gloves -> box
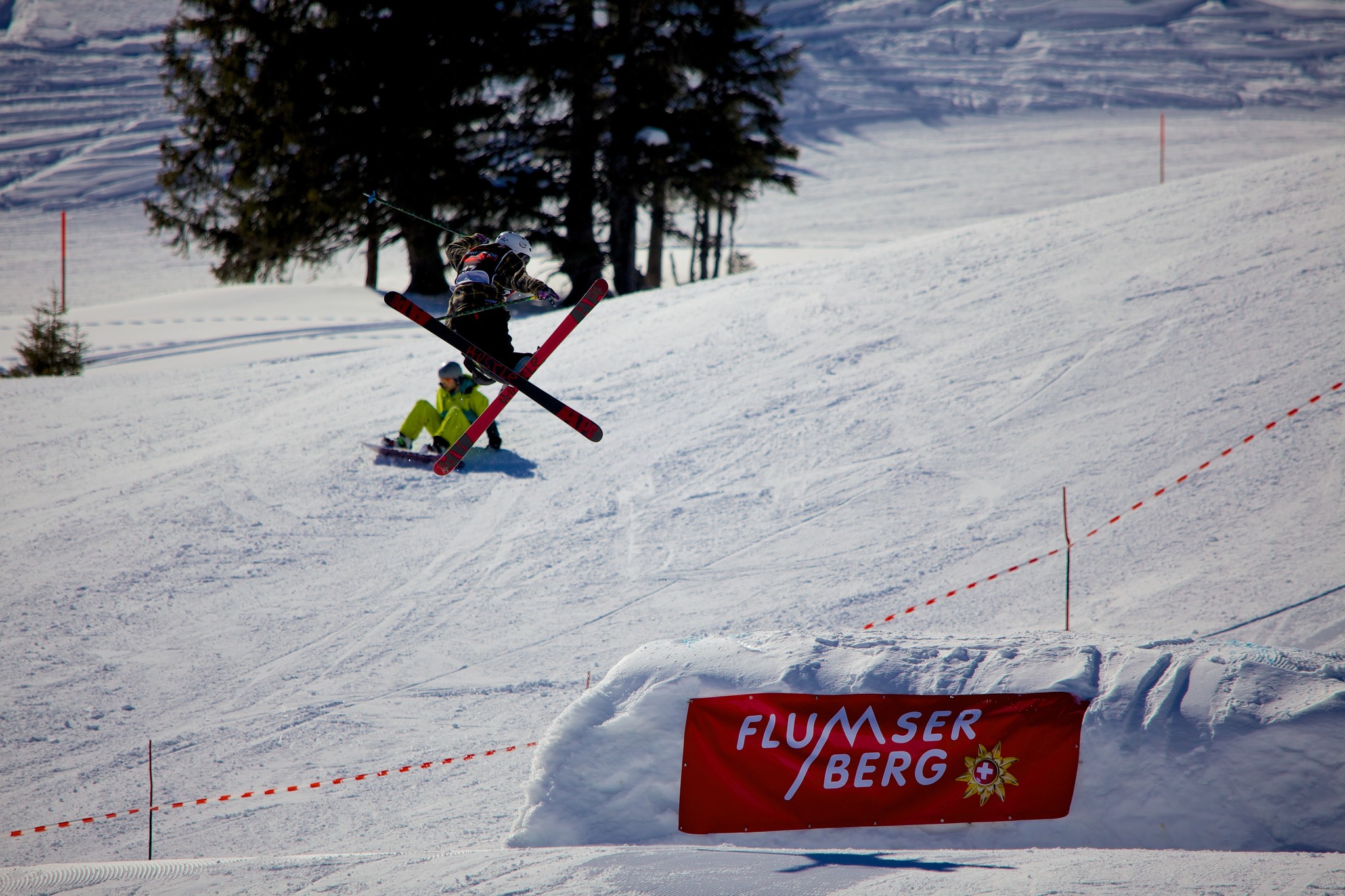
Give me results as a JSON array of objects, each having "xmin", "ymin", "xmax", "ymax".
[
  {"xmin": 473, "ymin": 232, "xmax": 489, "ymax": 244},
  {"xmin": 536, "ymin": 283, "xmax": 560, "ymax": 308},
  {"xmin": 489, "ymin": 437, "xmax": 502, "ymax": 449}
]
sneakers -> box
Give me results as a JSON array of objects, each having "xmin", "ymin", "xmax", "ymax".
[
  {"xmin": 419, "ymin": 444, "xmax": 445, "ymax": 456},
  {"xmin": 380, "ymin": 433, "xmax": 414, "ymax": 451}
]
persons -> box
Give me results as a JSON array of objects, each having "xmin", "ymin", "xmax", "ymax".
[
  {"xmin": 444, "ymin": 230, "xmax": 562, "ymax": 386},
  {"xmin": 381, "ymin": 361, "xmax": 503, "ymax": 455}
]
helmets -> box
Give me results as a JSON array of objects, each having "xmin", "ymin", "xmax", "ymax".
[
  {"xmin": 439, "ymin": 362, "xmax": 464, "ymax": 387},
  {"xmin": 494, "ymin": 231, "xmax": 534, "ymax": 265}
]
